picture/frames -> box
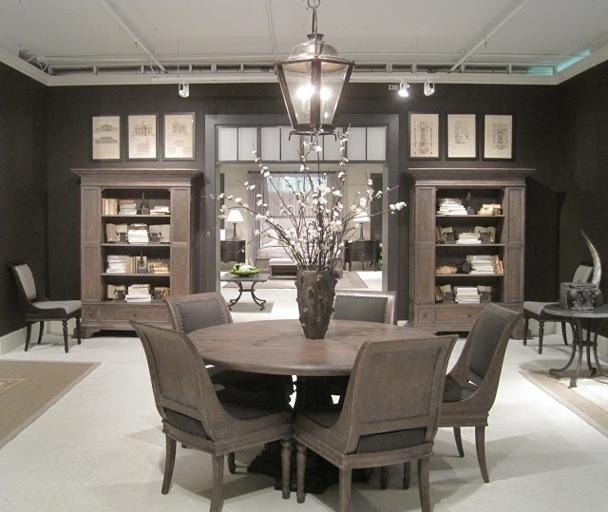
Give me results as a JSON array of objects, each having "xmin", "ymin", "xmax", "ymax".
[
  {"xmin": 90, "ymin": 111, "xmax": 197, "ymax": 162},
  {"xmin": 407, "ymin": 110, "xmax": 516, "ymax": 162}
]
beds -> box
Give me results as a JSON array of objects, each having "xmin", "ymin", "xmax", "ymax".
[{"xmin": 256, "ymin": 217, "xmax": 341, "ymax": 273}]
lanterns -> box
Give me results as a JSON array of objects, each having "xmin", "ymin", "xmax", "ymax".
[{"xmin": 272, "ymin": 33, "xmax": 355, "ymax": 143}]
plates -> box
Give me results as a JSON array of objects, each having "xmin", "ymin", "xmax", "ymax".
[{"xmin": 230, "ymin": 271, "xmax": 260, "ymax": 277}]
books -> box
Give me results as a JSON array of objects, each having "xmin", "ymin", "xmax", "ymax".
[
  {"xmin": 436, "ymin": 198, "xmax": 505, "ymax": 305},
  {"xmin": 102, "ymin": 197, "xmax": 170, "ymax": 303}
]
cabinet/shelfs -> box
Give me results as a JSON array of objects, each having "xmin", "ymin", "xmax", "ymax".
[
  {"xmin": 70, "ymin": 167, "xmax": 204, "ymax": 337},
  {"xmin": 405, "ymin": 164, "xmax": 536, "ymax": 341}
]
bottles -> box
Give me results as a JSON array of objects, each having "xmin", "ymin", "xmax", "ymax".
[{"xmin": 140, "ymin": 192, "xmax": 150, "ymax": 214}]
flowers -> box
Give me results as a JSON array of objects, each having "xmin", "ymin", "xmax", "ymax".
[{"xmin": 196, "ymin": 123, "xmax": 407, "ymax": 271}]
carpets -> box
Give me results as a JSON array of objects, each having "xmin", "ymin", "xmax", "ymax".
[
  {"xmin": 0, "ymin": 359, "xmax": 101, "ymax": 453},
  {"xmin": 223, "ymin": 271, "xmax": 368, "ymax": 289},
  {"xmin": 519, "ymin": 360, "xmax": 608, "ymax": 438}
]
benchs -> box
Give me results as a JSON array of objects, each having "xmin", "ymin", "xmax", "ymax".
[{"xmin": 268, "ymin": 258, "xmax": 342, "ymax": 280}]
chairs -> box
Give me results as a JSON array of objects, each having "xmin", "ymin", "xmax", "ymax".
[
  {"xmin": 130, "ymin": 320, "xmax": 292, "ymax": 512},
  {"xmin": 296, "ymin": 335, "xmax": 457, "ymax": 510},
  {"xmin": 401, "ymin": 302, "xmax": 520, "ymax": 487},
  {"xmin": 10, "ymin": 261, "xmax": 82, "ymax": 353},
  {"xmin": 166, "ymin": 292, "xmax": 233, "ymax": 388},
  {"xmin": 521, "ymin": 266, "xmax": 592, "ymax": 354},
  {"xmin": 332, "ymin": 290, "xmax": 396, "ymax": 323}
]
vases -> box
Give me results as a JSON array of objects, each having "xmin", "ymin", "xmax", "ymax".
[{"xmin": 295, "ymin": 269, "xmax": 339, "ymax": 339}]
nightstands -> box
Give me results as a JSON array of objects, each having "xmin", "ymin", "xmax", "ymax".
[
  {"xmin": 221, "ymin": 240, "xmax": 245, "ymax": 265},
  {"xmin": 345, "ymin": 240, "xmax": 379, "ymax": 271}
]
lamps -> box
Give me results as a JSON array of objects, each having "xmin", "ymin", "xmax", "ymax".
[
  {"xmin": 397, "ymin": 80, "xmax": 410, "ymax": 98},
  {"xmin": 352, "ymin": 211, "xmax": 371, "ymax": 240},
  {"xmin": 423, "ymin": 80, "xmax": 436, "ymax": 96},
  {"xmin": 226, "ymin": 208, "xmax": 245, "ymax": 240},
  {"xmin": 179, "ymin": 85, "xmax": 189, "ymax": 98},
  {"xmin": 272, "ymin": 1, "xmax": 355, "ymax": 143}
]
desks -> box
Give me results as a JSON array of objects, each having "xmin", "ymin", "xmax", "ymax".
[
  {"xmin": 185, "ymin": 319, "xmax": 437, "ymax": 491},
  {"xmin": 540, "ymin": 304, "xmax": 608, "ymax": 388},
  {"xmin": 220, "ymin": 271, "xmax": 270, "ymax": 310}
]
